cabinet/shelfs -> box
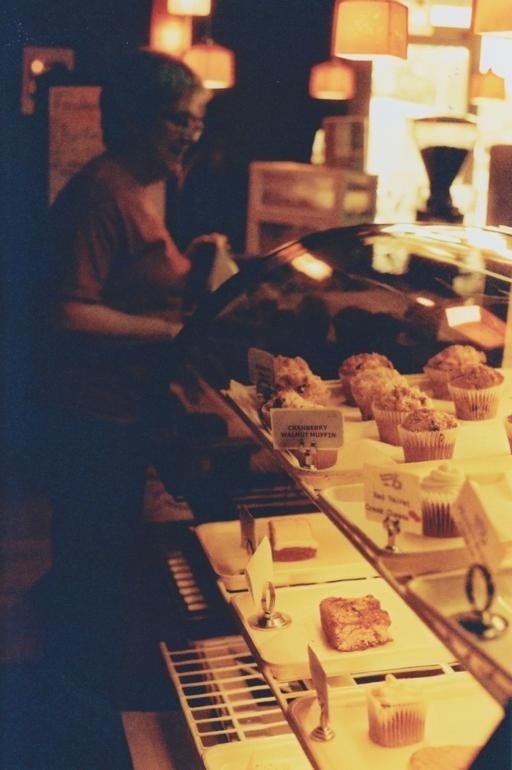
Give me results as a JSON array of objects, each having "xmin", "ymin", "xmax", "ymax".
[{"xmin": 106, "ymin": 217, "xmax": 512, "ymax": 770}]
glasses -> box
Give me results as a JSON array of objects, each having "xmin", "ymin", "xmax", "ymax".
[{"xmin": 171, "ymin": 113, "xmax": 204, "ymax": 137}]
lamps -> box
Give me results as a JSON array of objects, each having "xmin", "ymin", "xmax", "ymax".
[
  {"xmin": 413, "ymin": 115, "xmax": 476, "ymax": 223},
  {"xmin": 470, "ymin": 0, "xmax": 512, "ymax": 37},
  {"xmin": 469, "ymin": 69, "xmax": 507, "ymax": 104},
  {"xmin": 331, "ymin": 2, "xmax": 412, "ymax": 59},
  {"xmin": 308, "ymin": 60, "xmax": 362, "ymax": 99},
  {"xmin": 149, "ymin": 0, "xmax": 236, "ymax": 90}
]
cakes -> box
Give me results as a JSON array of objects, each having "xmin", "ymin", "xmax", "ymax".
[
  {"xmin": 369, "ymin": 673, "xmax": 425, "ymax": 749},
  {"xmin": 268, "ymin": 517, "xmax": 319, "ymax": 562},
  {"xmin": 420, "ymin": 464, "xmax": 480, "ymax": 539},
  {"xmin": 319, "ymin": 596, "xmax": 392, "ymax": 651},
  {"xmin": 272, "ymin": 343, "xmax": 512, "ymax": 470}
]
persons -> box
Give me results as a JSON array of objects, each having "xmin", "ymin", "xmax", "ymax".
[{"xmin": 32, "ymin": 47, "xmax": 233, "ymax": 683}]
